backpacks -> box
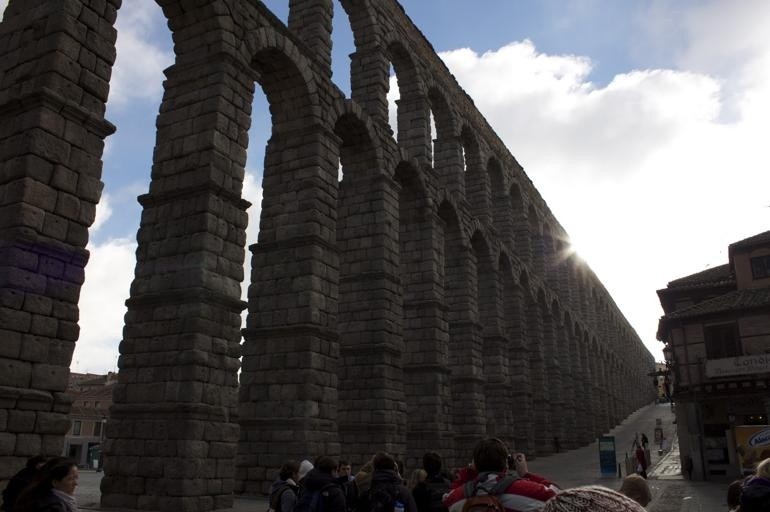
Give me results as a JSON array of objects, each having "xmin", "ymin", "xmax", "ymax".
[
  {"xmin": 269, "ymin": 477, "xmax": 450, "ymax": 512},
  {"xmin": 461, "ymin": 475, "xmax": 522, "ymax": 511}
]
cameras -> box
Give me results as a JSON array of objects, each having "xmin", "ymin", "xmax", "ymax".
[{"xmin": 506, "ymin": 455, "xmax": 516, "ymax": 470}]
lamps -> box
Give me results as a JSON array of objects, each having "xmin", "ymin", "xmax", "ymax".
[{"xmin": 662, "ymin": 343, "xmax": 678, "ymax": 369}]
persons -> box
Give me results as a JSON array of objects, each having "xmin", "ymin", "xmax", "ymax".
[
  {"xmin": 725, "ymin": 458, "xmax": 770, "ymax": 512},
  {"xmin": 267, "ymin": 439, "xmax": 648, "ymax": 511},
  {"xmin": 640, "ymin": 433, "xmax": 648, "ymax": 451},
  {"xmin": 3, "ymin": 455, "xmax": 79, "ymax": 512}
]
